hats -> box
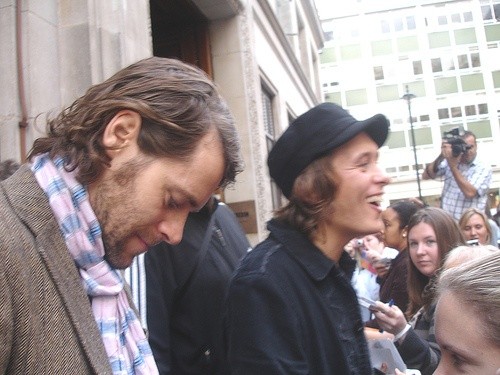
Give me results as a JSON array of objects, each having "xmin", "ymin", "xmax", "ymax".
[{"xmin": 267, "ymin": 103, "xmax": 390, "ymax": 199}]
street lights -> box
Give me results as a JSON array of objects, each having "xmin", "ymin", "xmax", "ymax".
[{"xmin": 401, "ymin": 84, "xmax": 425, "ymax": 204}]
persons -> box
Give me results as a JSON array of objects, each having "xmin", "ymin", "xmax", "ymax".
[
  {"xmin": 228, "ymin": 102, "xmax": 390, "ymax": 375},
  {"xmin": 0, "ymin": 57, "xmax": 244, "ymax": 375},
  {"xmin": 116, "ymin": 199, "xmax": 253, "ymax": 375},
  {"xmin": 422, "ymin": 129, "xmax": 491, "ymax": 227},
  {"xmin": 347, "ymin": 196, "xmax": 500, "ymax": 375},
  {"xmin": 0, "ymin": 158, "xmax": 20, "ymax": 182}
]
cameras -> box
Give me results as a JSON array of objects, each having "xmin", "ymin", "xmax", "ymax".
[{"xmin": 442, "ymin": 126, "xmax": 471, "ymax": 156}]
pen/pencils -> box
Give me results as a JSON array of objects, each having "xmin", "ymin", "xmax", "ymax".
[{"xmin": 378, "ymin": 298, "xmax": 395, "ymax": 333}]
